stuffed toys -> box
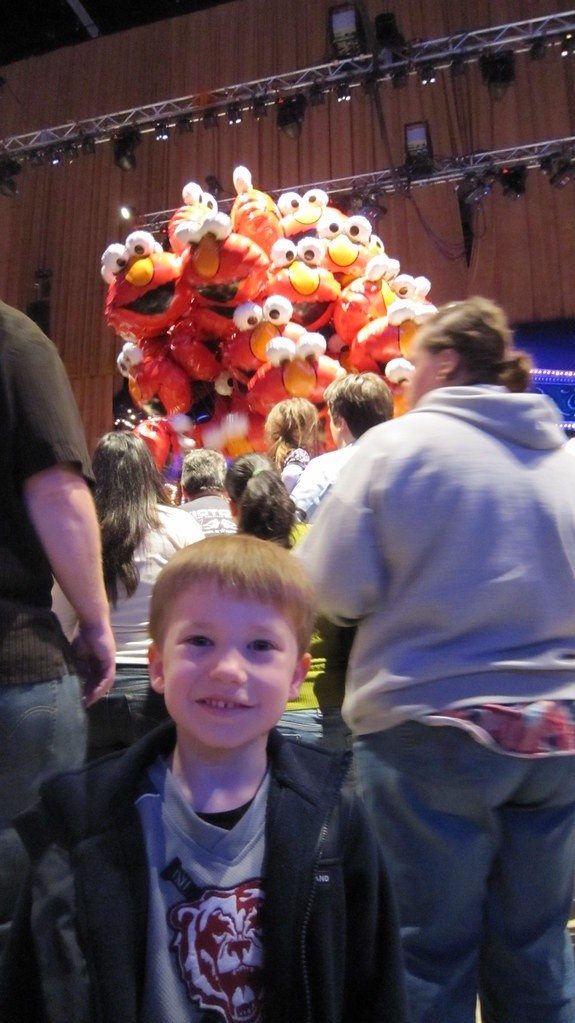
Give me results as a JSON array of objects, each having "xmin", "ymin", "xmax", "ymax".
[{"xmin": 101, "ymin": 163, "xmax": 440, "ymax": 495}]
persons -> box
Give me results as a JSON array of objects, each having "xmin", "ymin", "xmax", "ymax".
[
  {"xmin": 0, "ymin": 297, "xmax": 118, "ymax": 1023},
  {"xmin": 34, "ymin": 529, "xmax": 402, "ymax": 1023},
  {"xmin": 72, "ymin": 295, "xmax": 403, "ymax": 786},
  {"xmin": 293, "ymin": 293, "xmax": 575, "ymax": 1023}
]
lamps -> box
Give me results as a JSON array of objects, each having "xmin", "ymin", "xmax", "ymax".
[
  {"xmin": 362, "ymin": 70, "xmax": 409, "ymax": 95},
  {"xmin": 356, "ymin": 200, "xmax": 388, "ymax": 224},
  {"xmin": 478, "ymin": 50, "xmax": 516, "ymax": 102},
  {"xmin": 529, "ymin": 42, "xmax": 546, "ymax": 61},
  {"xmin": 275, "ymin": 94, "xmax": 307, "ymax": 142},
  {"xmin": 456, "ymin": 174, "xmax": 492, "ymax": 206},
  {"xmin": 0, "ymin": 154, "xmax": 22, "ymax": 197},
  {"xmin": 539, "ymin": 152, "xmax": 575, "ymax": 190},
  {"xmin": 449, "ymin": 59, "xmax": 466, "ymax": 81},
  {"xmin": 337, "ymin": 84, "xmax": 351, "ymax": 102},
  {"xmin": 28, "ymin": 137, "xmax": 95, "ymax": 168},
  {"xmin": 112, "ymin": 129, "xmax": 143, "ymax": 173},
  {"xmin": 154, "ymin": 99, "xmax": 268, "ymax": 141},
  {"xmin": 419, "ymin": 67, "xmax": 437, "ymax": 86}
]
